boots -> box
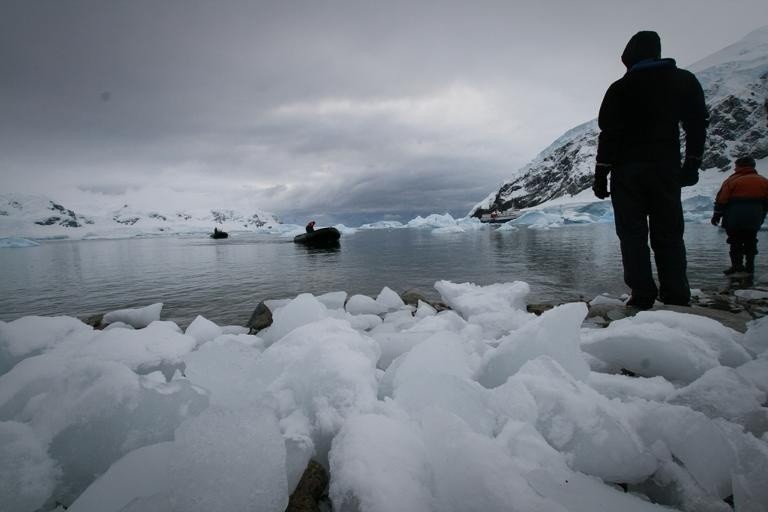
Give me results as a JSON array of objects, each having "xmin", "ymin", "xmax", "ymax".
[{"xmin": 723, "ymin": 251, "xmax": 754, "ymax": 274}]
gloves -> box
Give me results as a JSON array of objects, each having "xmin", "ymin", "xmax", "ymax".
[
  {"xmin": 681, "ymin": 156, "xmax": 699, "ymax": 186},
  {"xmin": 711, "ymin": 212, "xmax": 722, "ymax": 226},
  {"xmin": 592, "ymin": 164, "xmax": 612, "ymax": 199}
]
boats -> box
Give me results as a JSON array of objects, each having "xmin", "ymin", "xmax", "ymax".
[
  {"xmin": 209, "ymin": 231, "xmax": 229, "ymax": 239},
  {"xmin": 294, "ymin": 226, "xmax": 342, "ymax": 244},
  {"xmin": 479, "ymin": 198, "xmax": 522, "ymax": 224}
]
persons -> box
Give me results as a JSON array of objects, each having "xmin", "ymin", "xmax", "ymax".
[
  {"xmin": 592, "ymin": 31, "xmax": 706, "ymax": 309},
  {"xmin": 711, "ymin": 157, "xmax": 768, "ymax": 274},
  {"xmin": 306, "ymin": 221, "xmax": 315, "ymax": 233}
]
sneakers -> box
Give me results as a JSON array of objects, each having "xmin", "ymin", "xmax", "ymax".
[{"xmin": 626, "ymin": 288, "xmax": 690, "ymax": 308}]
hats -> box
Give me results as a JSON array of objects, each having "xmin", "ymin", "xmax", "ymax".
[
  {"xmin": 735, "ymin": 157, "xmax": 756, "ymax": 169},
  {"xmin": 621, "ymin": 31, "xmax": 661, "ymax": 61}
]
